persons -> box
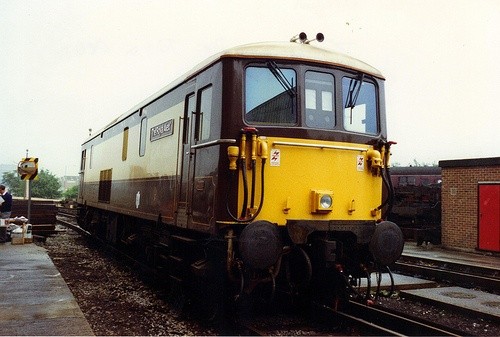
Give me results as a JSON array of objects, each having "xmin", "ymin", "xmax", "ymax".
[{"xmin": 0, "ymin": 184, "xmax": 12, "ymax": 241}]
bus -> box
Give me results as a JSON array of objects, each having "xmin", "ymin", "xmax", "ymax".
[{"xmin": 77, "ymin": 32, "xmax": 401, "ymax": 309}]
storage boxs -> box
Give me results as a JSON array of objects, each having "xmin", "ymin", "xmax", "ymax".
[
  {"xmin": 11, "ymin": 234, "xmax": 23, "ymax": 245},
  {"xmin": 25, "ymin": 238, "xmax": 33, "ymax": 243}
]
